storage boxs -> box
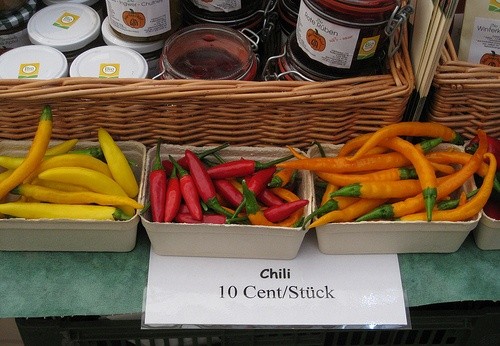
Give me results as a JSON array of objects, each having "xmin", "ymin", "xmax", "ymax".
[
  {"xmin": 0, "ymin": 139, "xmax": 148, "ymax": 254},
  {"xmin": 0, "ymin": 0, "xmax": 499, "ymax": 149},
  {"xmin": 305, "ymin": 142, "xmax": 482, "ymax": 255},
  {"xmin": 137, "ymin": 142, "xmax": 313, "ymax": 261},
  {"xmin": 462, "ymin": 142, "xmax": 500, "ymax": 251}
]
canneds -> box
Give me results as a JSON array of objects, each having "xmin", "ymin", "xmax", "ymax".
[{"xmin": 1, "ymin": 0, "xmax": 414, "ymax": 82}]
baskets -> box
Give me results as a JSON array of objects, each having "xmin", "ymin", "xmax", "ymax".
[
  {"xmin": 0, "ymin": 1, "xmax": 415, "ymax": 147},
  {"xmin": 425, "ymin": 30, "xmax": 500, "ymax": 144}
]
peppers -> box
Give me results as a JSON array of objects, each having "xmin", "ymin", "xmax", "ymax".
[{"xmin": 0, "ymin": 105, "xmax": 500, "ymax": 230}]
